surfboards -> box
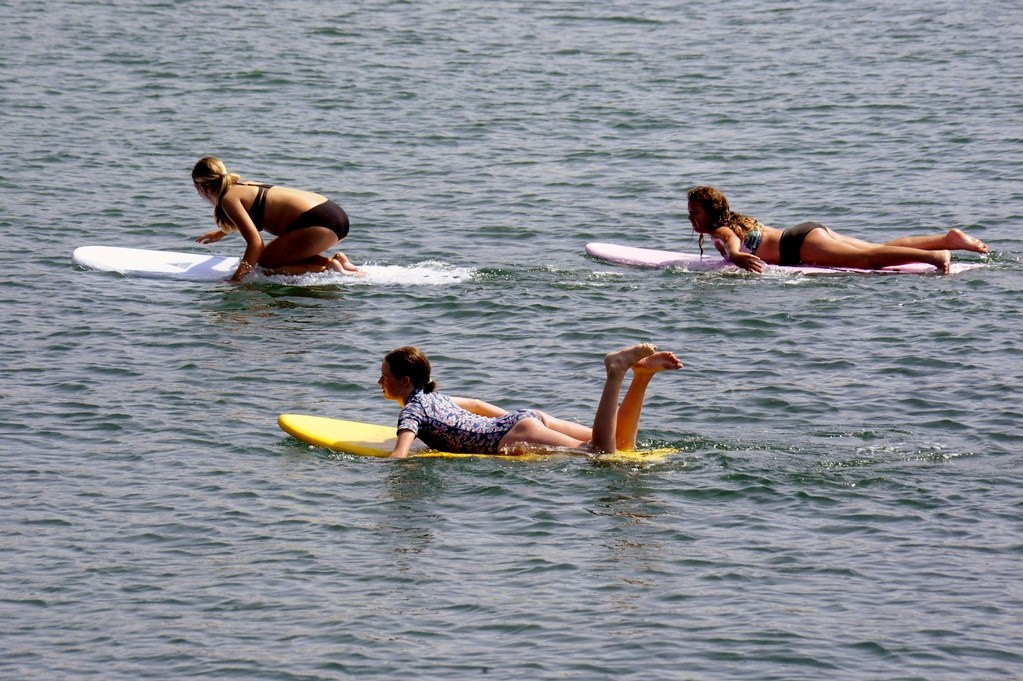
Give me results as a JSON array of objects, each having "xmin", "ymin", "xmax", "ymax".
[
  {"xmin": 278, "ymin": 412, "xmax": 679, "ymax": 463},
  {"xmin": 584, "ymin": 240, "xmax": 991, "ymax": 274},
  {"xmin": 73, "ymin": 244, "xmax": 465, "ymax": 284}
]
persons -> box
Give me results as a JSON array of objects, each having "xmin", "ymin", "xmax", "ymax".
[
  {"xmin": 687, "ymin": 187, "xmax": 988, "ymax": 274},
  {"xmin": 192, "ymin": 157, "xmax": 359, "ymax": 283},
  {"xmin": 378, "ymin": 345, "xmax": 682, "ymax": 460}
]
